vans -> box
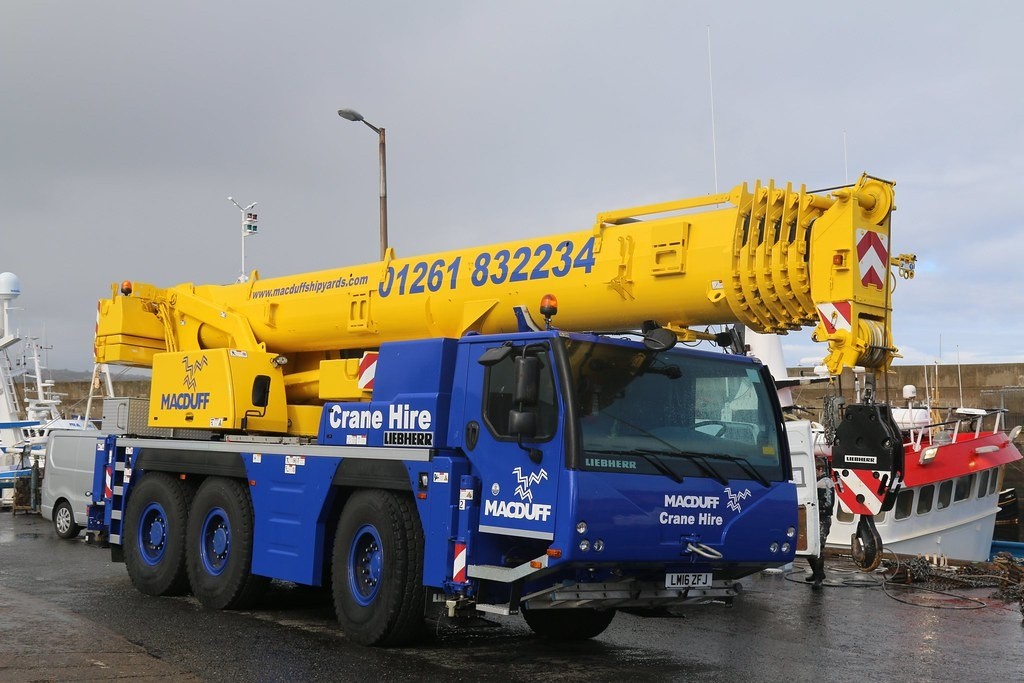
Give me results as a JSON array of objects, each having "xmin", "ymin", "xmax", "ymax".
[{"xmin": 41, "ymin": 429, "xmax": 107, "ymax": 539}]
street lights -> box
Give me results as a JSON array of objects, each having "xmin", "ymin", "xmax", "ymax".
[
  {"xmin": 337, "ymin": 108, "xmax": 388, "ymax": 263},
  {"xmin": 229, "ymin": 197, "xmax": 258, "ymax": 283}
]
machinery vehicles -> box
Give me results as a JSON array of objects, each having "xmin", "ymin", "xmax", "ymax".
[{"xmin": 90, "ymin": 173, "xmax": 904, "ymax": 652}]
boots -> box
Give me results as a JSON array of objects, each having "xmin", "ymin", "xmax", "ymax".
[
  {"xmin": 812, "ymin": 557, "xmax": 824, "ymax": 589},
  {"xmin": 806, "ymin": 555, "xmax": 826, "ymax": 581}
]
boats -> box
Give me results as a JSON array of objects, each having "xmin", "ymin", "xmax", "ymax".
[
  {"xmin": 0, "ymin": 272, "xmax": 103, "ymax": 502},
  {"xmin": 694, "ymin": 329, "xmax": 1023, "ymax": 565}
]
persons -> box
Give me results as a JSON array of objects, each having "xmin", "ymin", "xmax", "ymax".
[{"xmin": 805, "ymin": 462, "xmax": 834, "ymax": 590}]
